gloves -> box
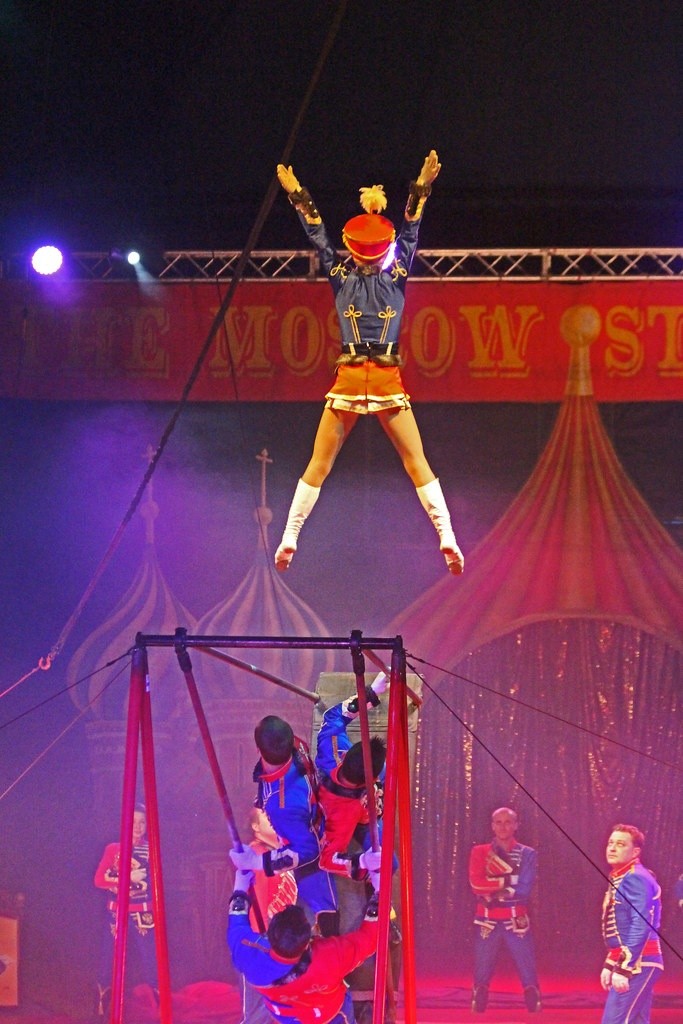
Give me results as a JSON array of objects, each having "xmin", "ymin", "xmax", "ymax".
[
  {"xmin": 360, "ymin": 845, "xmax": 381, "ymax": 872},
  {"xmin": 371, "ymin": 666, "xmax": 392, "ymax": 695},
  {"xmin": 234, "ymin": 869, "xmax": 256, "ymax": 893},
  {"xmin": 368, "ymin": 869, "xmax": 380, "ymax": 891},
  {"xmin": 228, "ymin": 843, "xmax": 263, "ymax": 872},
  {"xmin": 611, "ymin": 971, "xmax": 630, "ymax": 993},
  {"xmin": 600, "ymin": 967, "xmax": 613, "ymax": 992}
]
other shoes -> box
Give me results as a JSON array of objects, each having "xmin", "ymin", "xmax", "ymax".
[{"xmin": 389, "ymin": 920, "xmax": 403, "ymax": 946}]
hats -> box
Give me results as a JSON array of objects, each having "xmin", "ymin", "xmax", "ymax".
[{"xmin": 342, "ymin": 184, "xmax": 396, "ymax": 264}]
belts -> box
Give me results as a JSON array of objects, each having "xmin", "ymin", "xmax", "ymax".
[{"xmin": 342, "ymin": 343, "xmax": 397, "ymax": 356}]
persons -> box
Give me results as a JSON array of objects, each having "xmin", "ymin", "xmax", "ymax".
[
  {"xmin": 246, "ymin": 713, "xmax": 338, "ymax": 940},
  {"xmin": 599, "ymin": 822, "xmax": 664, "ymax": 1024},
  {"xmin": 312, "ymin": 663, "xmax": 403, "ymax": 947},
  {"xmin": 468, "ymin": 806, "xmax": 544, "ymax": 1015},
  {"xmin": 226, "ymin": 868, "xmax": 385, "ymax": 1024},
  {"xmin": 273, "ymin": 147, "xmax": 464, "ymax": 574},
  {"xmin": 93, "ymin": 801, "xmax": 164, "ymax": 1024}
]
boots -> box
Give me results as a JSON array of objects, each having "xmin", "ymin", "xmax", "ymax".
[
  {"xmin": 274, "ymin": 478, "xmax": 321, "ymax": 572},
  {"xmin": 415, "ymin": 478, "xmax": 465, "ymax": 576}
]
